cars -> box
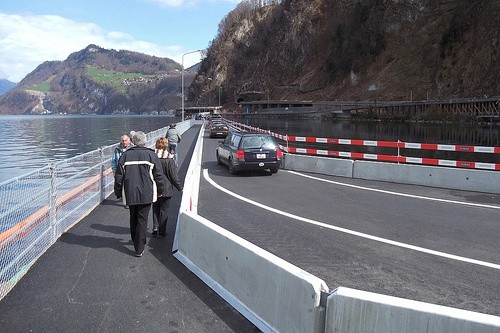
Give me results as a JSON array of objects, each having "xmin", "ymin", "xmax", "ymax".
[
  {"xmin": 215, "ymin": 130, "xmax": 283, "ymax": 174},
  {"xmin": 185, "ymin": 113, "xmax": 229, "ymax": 138}
]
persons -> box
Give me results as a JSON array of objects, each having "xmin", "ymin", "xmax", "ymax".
[{"xmin": 112, "ymin": 123, "xmax": 184, "ymax": 257}]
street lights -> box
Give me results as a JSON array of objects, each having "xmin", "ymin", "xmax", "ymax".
[
  {"xmin": 182, "ymin": 49, "xmax": 204, "ymax": 132},
  {"xmin": 197, "ymin": 65, "xmax": 220, "ymax": 113}
]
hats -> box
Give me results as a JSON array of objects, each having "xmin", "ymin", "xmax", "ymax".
[{"xmin": 170, "ymin": 123, "xmax": 177, "ymax": 127}]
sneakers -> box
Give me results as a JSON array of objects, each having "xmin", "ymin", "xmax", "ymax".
[{"xmin": 135, "ymin": 249, "xmax": 144, "ymax": 256}]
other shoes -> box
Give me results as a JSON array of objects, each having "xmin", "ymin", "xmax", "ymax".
[
  {"xmin": 153, "ymin": 231, "xmax": 157, "ymax": 235},
  {"xmin": 126, "ymin": 205, "xmax": 129, "ymax": 209},
  {"xmin": 116, "ymin": 196, "xmax": 121, "ymax": 201},
  {"xmin": 157, "ymin": 233, "xmax": 167, "ymax": 239}
]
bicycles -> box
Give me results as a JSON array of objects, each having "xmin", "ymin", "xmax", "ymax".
[{"xmin": 170, "ymin": 139, "xmax": 181, "ymax": 156}]
